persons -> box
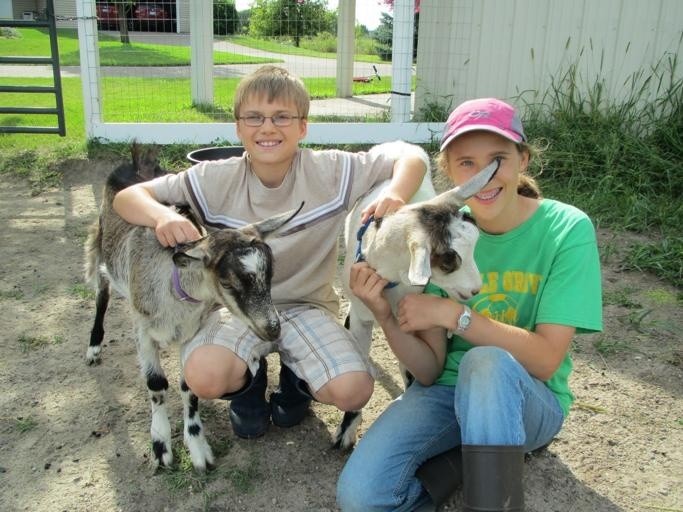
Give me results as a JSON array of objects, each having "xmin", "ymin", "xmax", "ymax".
[
  {"xmin": 110, "ymin": 63, "xmax": 429, "ymax": 441},
  {"xmin": 335, "ymin": 96, "xmax": 605, "ymax": 510}
]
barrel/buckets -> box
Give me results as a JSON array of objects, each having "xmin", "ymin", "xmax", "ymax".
[{"xmin": 186, "ymin": 146, "xmax": 245, "ymax": 166}]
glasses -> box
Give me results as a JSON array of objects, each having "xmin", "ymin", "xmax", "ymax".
[{"xmin": 239, "ymin": 112, "xmax": 299, "ymax": 127}]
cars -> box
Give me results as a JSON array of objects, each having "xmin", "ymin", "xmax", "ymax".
[{"xmin": 96, "ymin": 3, "xmax": 170, "ymax": 31}]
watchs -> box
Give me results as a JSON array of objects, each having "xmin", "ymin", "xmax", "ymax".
[{"xmin": 446, "ymin": 304, "xmax": 473, "ymax": 341}]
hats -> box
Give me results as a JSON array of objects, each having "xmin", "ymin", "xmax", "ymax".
[{"xmin": 439, "ymin": 98, "xmax": 527, "ymax": 152}]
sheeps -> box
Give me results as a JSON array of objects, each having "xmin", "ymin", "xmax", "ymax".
[
  {"xmin": 82, "ymin": 136, "xmax": 305, "ymax": 476},
  {"xmin": 325, "ymin": 157, "xmax": 503, "ymax": 464}
]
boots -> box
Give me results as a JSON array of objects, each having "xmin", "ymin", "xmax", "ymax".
[
  {"xmin": 412, "ymin": 443, "xmax": 525, "ymax": 512},
  {"xmin": 220, "ymin": 355, "xmax": 272, "ymax": 439},
  {"xmin": 270, "ymin": 359, "xmax": 318, "ymax": 428}
]
